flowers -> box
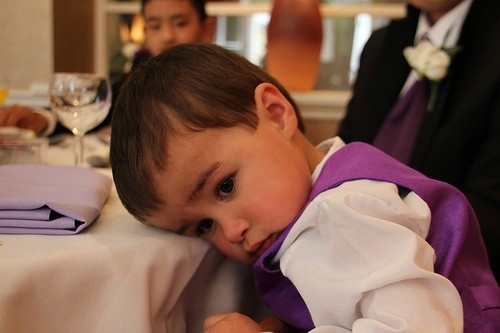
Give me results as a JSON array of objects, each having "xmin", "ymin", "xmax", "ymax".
[{"xmin": 402, "ymin": 38, "xmax": 462, "ymax": 113}]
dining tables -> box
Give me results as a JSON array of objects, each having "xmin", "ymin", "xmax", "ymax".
[{"xmin": 0, "ymin": 127, "xmax": 254, "ymax": 333}]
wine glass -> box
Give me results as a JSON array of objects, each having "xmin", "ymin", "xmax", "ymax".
[{"xmin": 49, "ymin": 72, "xmax": 111, "ymax": 165}]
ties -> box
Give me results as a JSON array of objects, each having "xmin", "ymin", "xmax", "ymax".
[{"xmin": 372, "ymin": 34, "xmax": 432, "ymax": 166}]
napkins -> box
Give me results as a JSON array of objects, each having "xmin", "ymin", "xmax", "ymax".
[{"xmin": 0, "ymin": 165, "xmax": 112, "ymax": 235}]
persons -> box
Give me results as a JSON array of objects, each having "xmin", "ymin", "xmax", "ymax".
[
  {"xmin": 332, "ymin": 0, "xmax": 500, "ymax": 286},
  {"xmin": 0, "ymin": 0, "xmax": 218, "ymax": 137},
  {"xmin": 108, "ymin": 41, "xmax": 500, "ymax": 333}
]
